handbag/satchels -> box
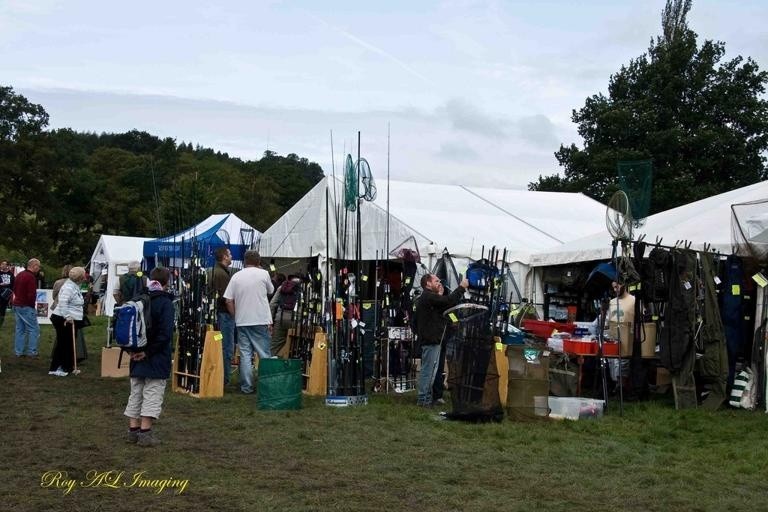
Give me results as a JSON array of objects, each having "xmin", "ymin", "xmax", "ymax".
[
  {"xmin": 466, "ymin": 263, "xmax": 499, "ymax": 292},
  {"xmin": 77, "ymin": 316, "xmax": 90, "ymax": 329}
]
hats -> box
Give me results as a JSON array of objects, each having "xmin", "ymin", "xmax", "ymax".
[{"xmin": 288, "ymin": 272, "xmax": 308, "ymax": 284}]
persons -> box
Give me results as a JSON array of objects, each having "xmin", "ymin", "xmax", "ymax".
[
  {"xmin": 413, "ymin": 274, "xmax": 470, "ymax": 408},
  {"xmin": 436, "ymin": 280, "xmax": 452, "ymax": 405},
  {"xmin": 1, "ymin": 247, "xmax": 307, "ymax": 448},
  {"xmin": 606, "ymin": 279, "xmax": 646, "ymax": 398}
]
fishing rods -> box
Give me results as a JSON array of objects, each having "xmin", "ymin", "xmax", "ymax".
[
  {"xmin": 262, "ymin": 201, "xmax": 312, "ymax": 269},
  {"xmin": 154, "ymin": 164, "xmax": 220, "ymax": 394},
  {"xmin": 288, "ymin": 121, "xmax": 416, "ymax": 395},
  {"xmin": 448, "ymin": 245, "xmax": 513, "ymax": 422}
]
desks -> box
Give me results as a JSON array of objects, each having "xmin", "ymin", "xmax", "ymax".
[{"xmin": 576, "ymin": 353, "xmax": 702, "ymax": 415}]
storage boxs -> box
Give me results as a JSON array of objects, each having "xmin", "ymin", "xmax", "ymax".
[{"xmin": 520, "ymin": 319, "xmax": 618, "ymax": 422}]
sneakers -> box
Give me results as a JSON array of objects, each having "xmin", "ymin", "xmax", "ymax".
[
  {"xmin": 419, "ymin": 397, "xmax": 446, "ymax": 410},
  {"xmin": 48, "ymin": 369, "xmax": 81, "ymax": 377},
  {"xmin": 16, "ymin": 354, "xmax": 40, "ymax": 360},
  {"xmin": 127, "ymin": 428, "xmax": 162, "ymax": 447}
]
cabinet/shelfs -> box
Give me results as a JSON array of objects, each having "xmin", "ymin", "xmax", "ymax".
[
  {"xmin": 283, "ymin": 328, "xmax": 327, "ymax": 396},
  {"xmin": 171, "ymin": 323, "xmax": 224, "ymax": 399}
]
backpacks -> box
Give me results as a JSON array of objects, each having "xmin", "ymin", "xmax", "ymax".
[
  {"xmin": 280, "ymin": 280, "xmax": 299, "ymax": 311},
  {"xmin": 114, "ymin": 292, "xmax": 169, "ymax": 350}
]
